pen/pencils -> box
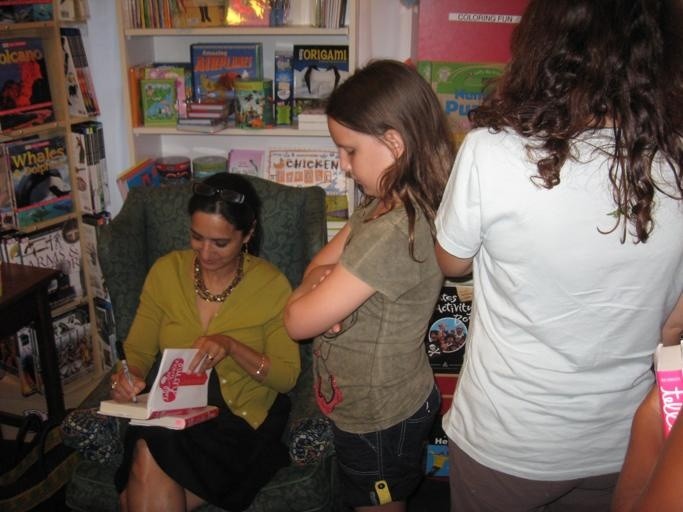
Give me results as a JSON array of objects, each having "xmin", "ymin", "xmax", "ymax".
[{"xmin": 116, "ymin": 340, "xmax": 138, "ymax": 402}]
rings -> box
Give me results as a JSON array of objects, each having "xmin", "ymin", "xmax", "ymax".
[
  {"xmin": 205, "ymin": 351, "xmax": 215, "ymax": 361},
  {"xmin": 197, "ymin": 349, "xmax": 205, "ymax": 358}
]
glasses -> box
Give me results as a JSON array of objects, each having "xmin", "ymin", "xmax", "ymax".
[{"xmin": 192, "ymin": 181, "xmax": 248, "ymax": 207}]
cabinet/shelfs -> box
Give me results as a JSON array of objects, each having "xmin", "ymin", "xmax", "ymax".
[
  {"xmin": 0, "ymin": 0, "xmax": 110, "ymax": 398},
  {"xmin": 114, "ymin": 1, "xmax": 357, "ymax": 244}
]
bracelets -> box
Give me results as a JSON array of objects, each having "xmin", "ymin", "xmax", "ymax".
[{"xmin": 246, "ymin": 352, "xmax": 266, "ymax": 382}]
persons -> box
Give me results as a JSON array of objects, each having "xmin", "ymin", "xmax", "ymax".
[
  {"xmin": 429, "ymin": 0, "xmax": 682, "ymax": 512},
  {"xmin": 606, "ymin": 347, "xmax": 682, "ymax": 512},
  {"xmin": 280, "ymin": 58, "xmax": 461, "ymax": 512},
  {"xmin": 100, "ymin": 170, "xmax": 302, "ymax": 511}
]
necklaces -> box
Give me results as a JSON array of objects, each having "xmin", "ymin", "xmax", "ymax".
[{"xmin": 186, "ymin": 252, "xmax": 245, "ymax": 305}]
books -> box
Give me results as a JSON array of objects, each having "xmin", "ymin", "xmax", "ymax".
[
  {"xmin": 414, "ymin": 0, "xmax": 528, "ymax": 152},
  {"xmin": 0, "ymin": 120, "xmax": 119, "ymax": 396},
  {"xmin": 0, "ymin": 0, "xmax": 90, "ymax": 24},
  {"xmin": 421, "ymin": 280, "xmax": 475, "ymax": 482},
  {"xmin": 117, "ymin": 158, "xmax": 160, "ymax": 199},
  {"xmin": 0, "ymin": 27, "xmax": 100, "ymax": 134},
  {"xmin": 227, "ymin": 149, "xmax": 348, "ymax": 222},
  {"xmin": 127, "ymin": 42, "xmax": 349, "ymax": 134},
  {"xmin": 93, "ymin": 343, "xmax": 219, "ymax": 420},
  {"xmin": 125, "ymin": 0, "xmax": 348, "ymax": 28},
  {"xmin": 125, "ymin": 404, "xmax": 217, "ymax": 431}
]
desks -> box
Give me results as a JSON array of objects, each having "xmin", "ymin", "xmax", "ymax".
[{"xmin": 1, "ymin": 262, "xmax": 64, "ymax": 431}]
